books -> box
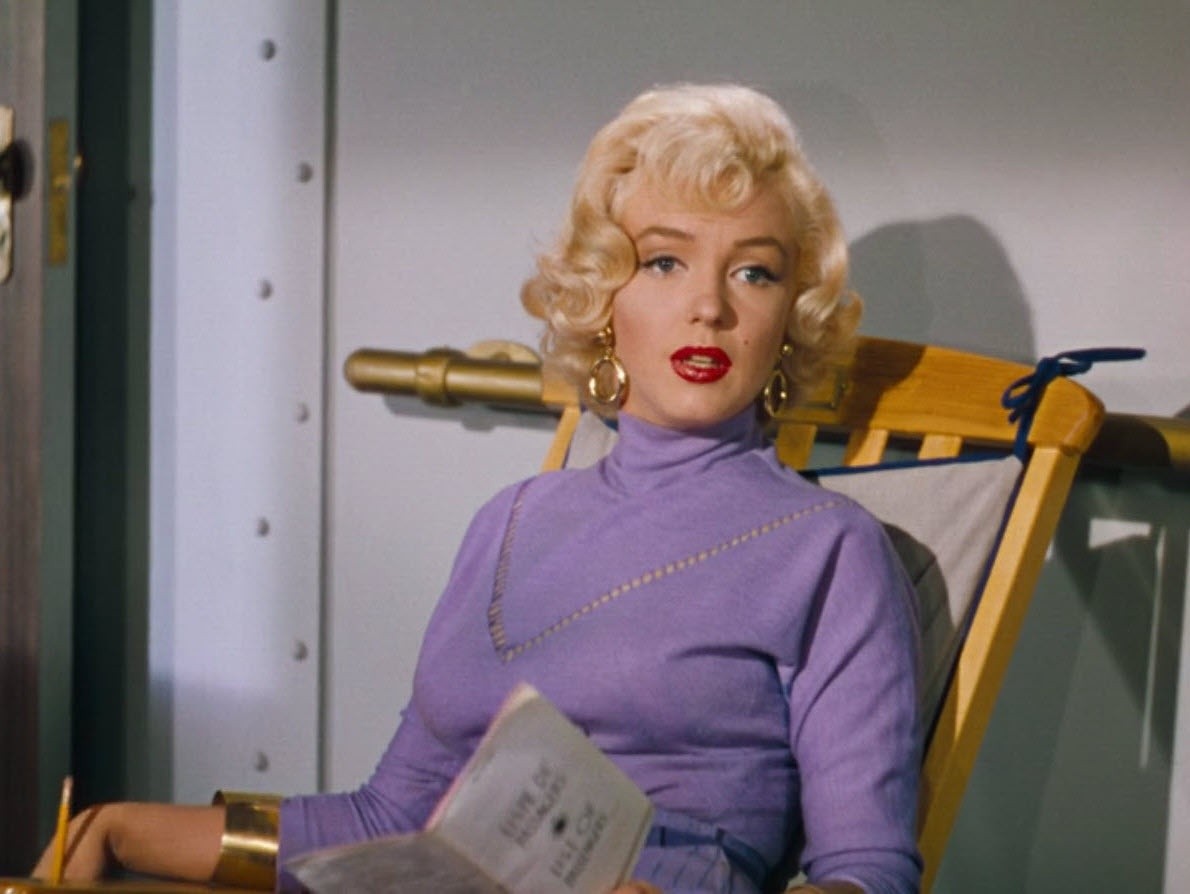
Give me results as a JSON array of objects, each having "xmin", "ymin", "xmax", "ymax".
[{"xmin": 280, "ymin": 682, "xmax": 657, "ymax": 893}]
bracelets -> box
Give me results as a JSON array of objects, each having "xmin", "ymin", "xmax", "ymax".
[{"xmin": 207, "ymin": 788, "xmax": 284, "ymax": 893}]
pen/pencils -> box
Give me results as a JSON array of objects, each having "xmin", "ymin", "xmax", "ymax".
[{"xmin": 51, "ymin": 777, "xmax": 74, "ymax": 888}]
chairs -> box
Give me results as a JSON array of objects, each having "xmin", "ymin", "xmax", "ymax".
[{"xmin": 0, "ymin": 334, "xmax": 1103, "ymax": 894}]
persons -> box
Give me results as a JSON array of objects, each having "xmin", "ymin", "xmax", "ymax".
[{"xmin": 32, "ymin": 83, "xmax": 928, "ymax": 894}]
